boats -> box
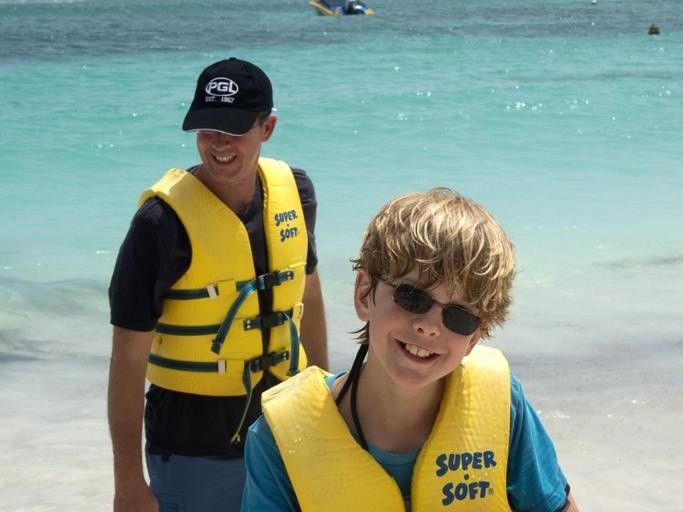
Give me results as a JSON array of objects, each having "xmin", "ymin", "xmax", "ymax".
[{"xmin": 308, "ymin": 0, "xmax": 375, "ymax": 17}]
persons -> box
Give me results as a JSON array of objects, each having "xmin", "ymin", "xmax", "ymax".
[
  {"xmin": 238, "ymin": 187, "xmax": 580, "ymax": 512},
  {"xmin": 105, "ymin": 57, "xmax": 329, "ymax": 510}
]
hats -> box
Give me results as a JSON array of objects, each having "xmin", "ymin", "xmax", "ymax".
[{"xmin": 182, "ymin": 57, "xmax": 273, "ymax": 137}]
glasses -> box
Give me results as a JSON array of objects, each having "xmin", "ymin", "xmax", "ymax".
[{"xmin": 378, "ymin": 277, "xmax": 481, "ymax": 336}]
